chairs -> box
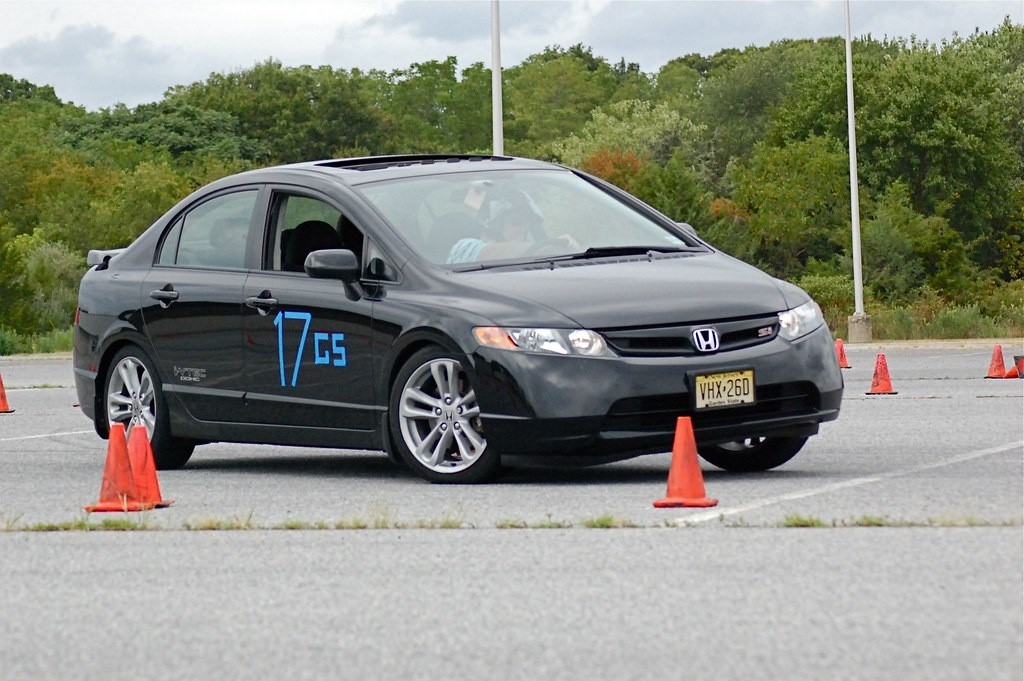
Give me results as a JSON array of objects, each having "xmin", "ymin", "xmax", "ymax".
[
  {"xmin": 196, "ymin": 215, "xmax": 363, "ymax": 273},
  {"xmin": 429, "ymin": 212, "xmax": 489, "ymax": 264}
]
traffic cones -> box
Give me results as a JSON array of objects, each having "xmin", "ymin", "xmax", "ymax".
[
  {"xmin": 866, "ymin": 353, "xmax": 900, "ymax": 395},
  {"xmin": 84, "ymin": 422, "xmax": 153, "ymax": 512},
  {"xmin": 123, "ymin": 426, "xmax": 175, "ymax": 510},
  {"xmin": 654, "ymin": 415, "xmax": 720, "ymax": 509},
  {"xmin": 1003, "ymin": 355, "xmax": 1024, "ymax": 380},
  {"xmin": 983, "ymin": 344, "xmax": 1007, "ymax": 379},
  {"xmin": 0, "ymin": 373, "xmax": 17, "ymax": 414},
  {"xmin": 834, "ymin": 338, "xmax": 853, "ymax": 369}
]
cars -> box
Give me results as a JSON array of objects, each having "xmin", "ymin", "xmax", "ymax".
[{"xmin": 71, "ymin": 149, "xmax": 846, "ymax": 485}]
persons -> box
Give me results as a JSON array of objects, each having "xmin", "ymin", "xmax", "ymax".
[{"xmin": 447, "ymin": 185, "xmax": 581, "ymax": 265}]
